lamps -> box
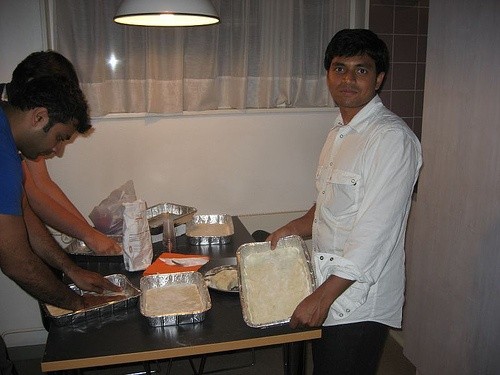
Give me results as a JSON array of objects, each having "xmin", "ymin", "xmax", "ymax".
[{"xmin": 111, "ymin": 0, "xmax": 222, "ymax": 29}]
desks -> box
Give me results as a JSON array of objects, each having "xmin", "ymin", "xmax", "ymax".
[{"xmin": 40, "ymin": 214, "xmax": 323, "ymax": 375}]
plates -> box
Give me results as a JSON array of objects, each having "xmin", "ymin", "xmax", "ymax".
[{"xmin": 205, "ymin": 265, "xmax": 240, "ymax": 293}]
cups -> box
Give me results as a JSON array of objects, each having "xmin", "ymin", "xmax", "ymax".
[{"xmin": 162, "ymin": 219, "xmax": 176, "ymax": 254}]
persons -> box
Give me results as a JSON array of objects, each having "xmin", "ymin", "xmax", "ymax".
[
  {"xmin": 0, "ymin": 49, "xmax": 123, "ymax": 257},
  {"xmin": 264, "ymin": 28, "xmax": 425, "ymax": 375},
  {"xmin": 0, "ymin": 72, "xmax": 125, "ymax": 375}
]
